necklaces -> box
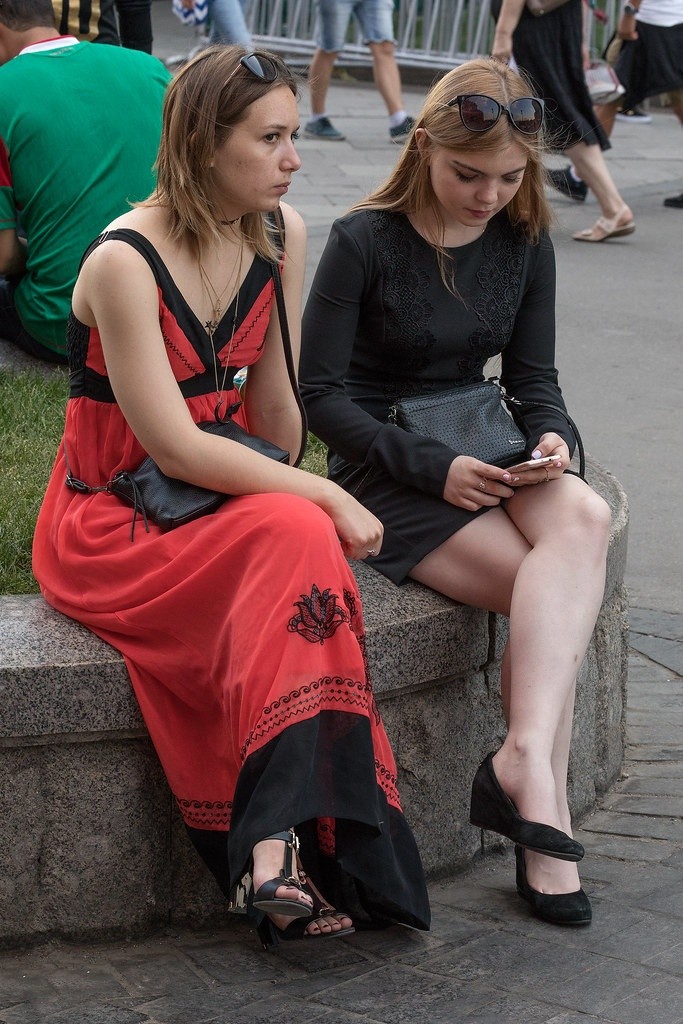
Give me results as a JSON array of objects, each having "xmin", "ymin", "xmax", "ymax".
[
  {"xmin": 198, "ymin": 232, "xmax": 244, "ymax": 424},
  {"xmin": 220, "ymin": 217, "xmax": 240, "ymax": 225}
]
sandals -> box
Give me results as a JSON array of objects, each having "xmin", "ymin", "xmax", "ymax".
[{"xmin": 571, "ymin": 205, "xmax": 635, "ymax": 242}]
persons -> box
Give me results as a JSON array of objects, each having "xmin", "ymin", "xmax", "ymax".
[
  {"xmin": 297, "ymin": 55, "xmax": 613, "ymax": 926},
  {"xmin": 31, "ymin": 43, "xmax": 432, "ymax": 950},
  {"xmin": 541, "ymin": 0, "xmax": 683, "ymax": 211},
  {"xmin": 490, "ymin": 0, "xmax": 636, "ymax": 242},
  {"xmin": 0, "ymin": 0, "xmax": 175, "ymax": 367},
  {"xmin": 172, "ymin": 0, "xmax": 253, "ymax": 47},
  {"xmin": 302, "ymin": 0, "xmax": 417, "ymax": 143}
]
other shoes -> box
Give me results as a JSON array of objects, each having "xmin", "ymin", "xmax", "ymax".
[
  {"xmin": 543, "ymin": 165, "xmax": 588, "ymax": 201},
  {"xmin": 663, "ymin": 193, "xmax": 683, "ymax": 209}
]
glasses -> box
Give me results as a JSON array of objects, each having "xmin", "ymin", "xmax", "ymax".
[
  {"xmin": 217, "ymin": 52, "xmax": 292, "ymax": 97},
  {"xmin": 434, "ymin": 92, "xmax": 545, "ymax": 136}
]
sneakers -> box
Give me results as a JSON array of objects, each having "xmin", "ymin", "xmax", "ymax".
[
  {"xmin": 614, "ymin": 106, "xmax": 652, "ymax": 124},
  {"xmin": 389, "ymin": 117, "xmax": 417, "ymax": 142},
  {"xmin": 303, "ymin": 116, "xmax": 346, "ymax": 141}
]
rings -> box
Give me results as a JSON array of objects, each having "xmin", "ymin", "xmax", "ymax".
[
  {"xmin": 479, "ymin": 479, "xmax": 487, "ymax": 492},
  {"xmin": 368, "ymin": 549, "xmax": 376, "ymax": 554},
  {"xmin": 542, "ymin": 467, "xmax": 549, "ymax": 483}
]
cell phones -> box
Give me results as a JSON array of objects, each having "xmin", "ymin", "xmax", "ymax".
[{"xmin": 504, "ymin": 455, "xmax": 562, "ymax": 473}]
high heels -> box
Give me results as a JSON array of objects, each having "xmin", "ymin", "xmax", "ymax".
[
  {"xmin": 254, "ymin": 868, "xmax": 357, "ymax": 951},
  {"xmin": 227, "ymin": 830, "xmax": 315, "ymax": 918},
  {"xmin": 514, "ymin": 843, "xmax": 593, "ymax": 926},
  {"xmin": 469, "ymin": 751, "xmax": 586, "ymax": 863}
]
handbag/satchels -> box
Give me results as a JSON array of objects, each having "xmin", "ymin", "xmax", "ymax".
[
  {"xmin": 108, "ymin": 421, "xmax": 290, "ymax": 544},
  {"xmin": 525, "ymin": 0, "xmax": 572, "ymax": 18},
  {"xmin": 172, "ymin": 0, "xmax": 209, "ymax": 26},
  {"xmin": 398, "ymin": 378, "xmax": 527, "ymax": 469},
  {"xmin": 582, "ymin": 58, "xmax": 625, "ymax": 105}
]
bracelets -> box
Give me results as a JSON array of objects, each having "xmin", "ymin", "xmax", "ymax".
[{"xmin": 624, "ymin": 4, "xmax": 638, "ymax": 15}]
